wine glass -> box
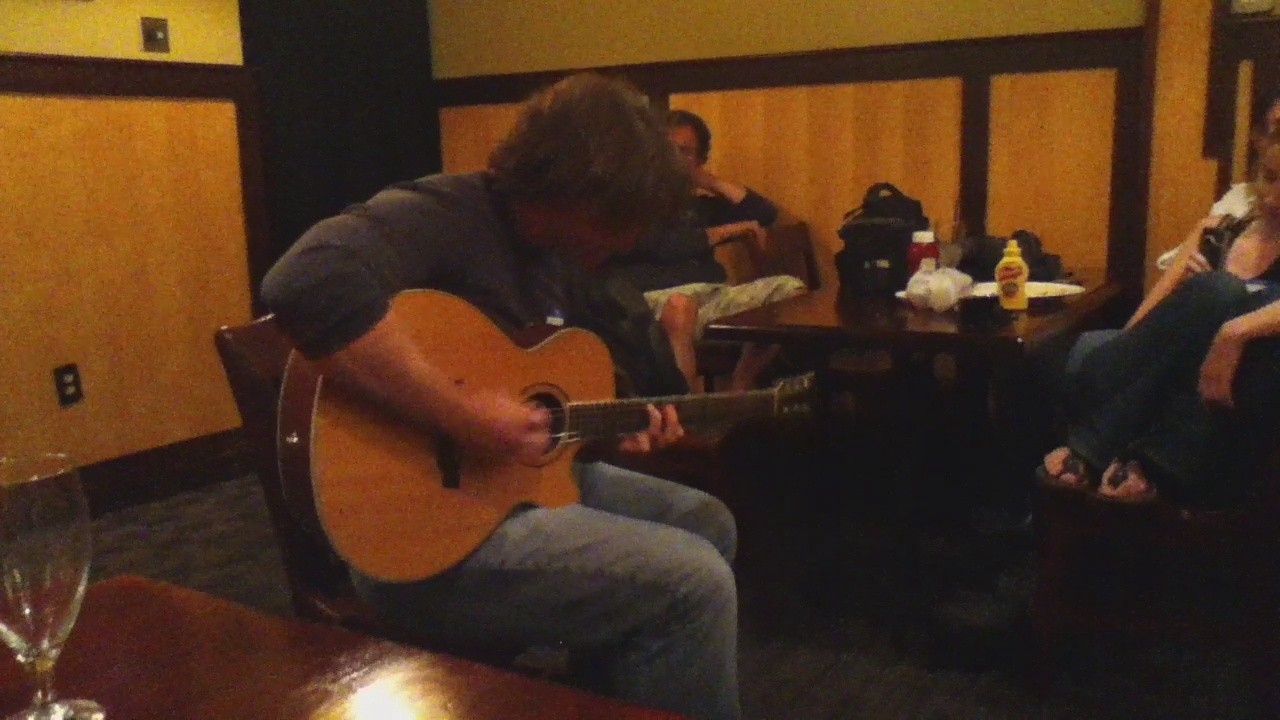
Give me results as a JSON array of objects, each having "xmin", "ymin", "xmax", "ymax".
[{"xmin": 0, "ymin": 453, "xmax": 104, "ymax": 720}]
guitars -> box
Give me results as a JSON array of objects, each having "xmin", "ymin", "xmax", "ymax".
[{"xmin": 274, "ymin": 288, "xmax": 819, "ymax": 583}]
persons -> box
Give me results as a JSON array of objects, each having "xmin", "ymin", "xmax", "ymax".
[
  {"xmin": 260, "ymin": 69, "xmax": 740, "ymax": 720},
  {"xmin": 623, "ymin": 108, "xmax": 780, "ymax": 388},
  {"xmin": 1033, "ymin": 97, "xmax": 1280, "ymax": 504}
]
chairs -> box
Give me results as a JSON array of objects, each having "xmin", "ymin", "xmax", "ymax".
[{"xmin": 215, "ymin": 314, "xmax": 606, "ymax": 685}]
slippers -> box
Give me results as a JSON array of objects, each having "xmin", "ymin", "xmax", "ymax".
[
  {"xmin": 1035, "ymin": 446, "xmax": 1092, "ymax": 493},
  {"xmin": 1097, "ymin": 458, "xmax": 1155, "ymax": 504}
]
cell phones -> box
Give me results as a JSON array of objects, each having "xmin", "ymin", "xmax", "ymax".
[{"xmin": 1200, "ymin": 228, "xmax": 1232, "ymax": 271}]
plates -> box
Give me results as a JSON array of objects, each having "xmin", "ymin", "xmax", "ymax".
[{"xmin": 894, "ymin": 280, "xmax": 1086, "ymax": 302}]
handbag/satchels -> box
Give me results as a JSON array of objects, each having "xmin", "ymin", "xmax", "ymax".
[{"xmin": 834, "ymin": 181, "xmax": 930, "ymax": 298}]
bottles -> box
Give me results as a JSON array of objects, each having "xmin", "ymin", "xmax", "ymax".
[
  {"xmin": 994, "ymin": 239, "xmax": 1029, "ymax": 310},
  {"xmin": 906, "ymin": 231, "xmax": 939, "ymax": 279}
]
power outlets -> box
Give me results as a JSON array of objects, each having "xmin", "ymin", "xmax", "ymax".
[{"xmin": 55, "ymin": 363, "xmax": 85, "ymax": 404}]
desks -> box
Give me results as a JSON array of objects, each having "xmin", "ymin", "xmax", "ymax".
[
  {"xmin": 0, "ymin": 581, "xmax": 673, "ymax": 719},
  {"xmin": 706, "ymin": 276, "xmax": 1120, "ymax": 397}
]
